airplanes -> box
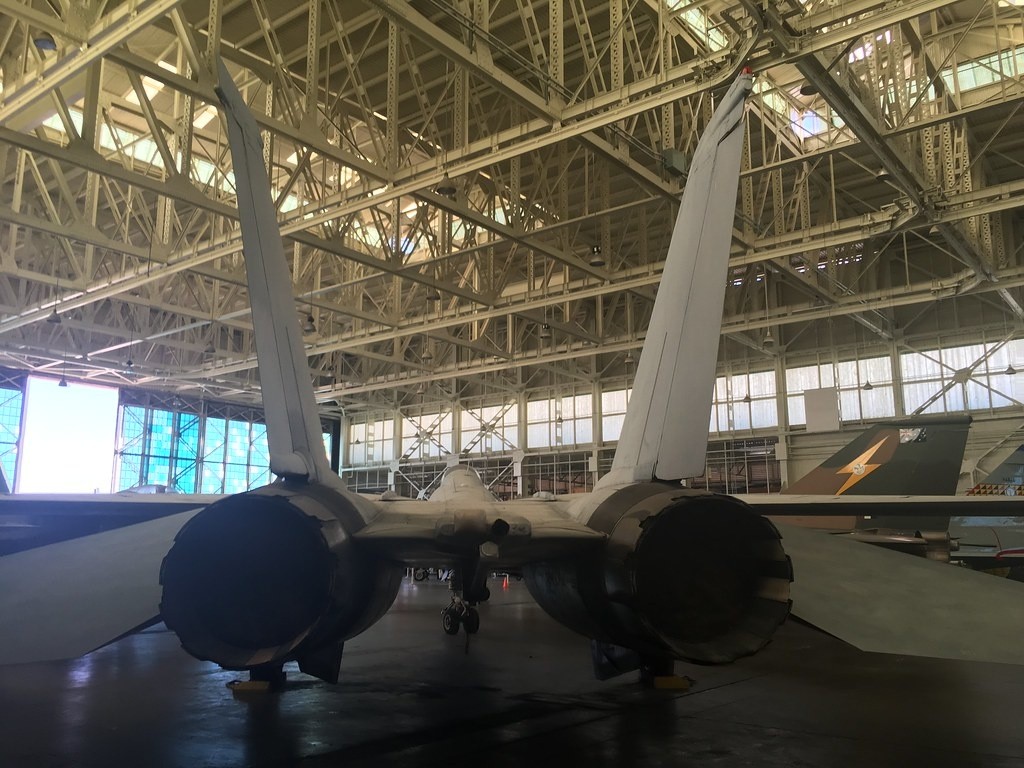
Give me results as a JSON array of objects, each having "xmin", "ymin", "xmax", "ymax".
[{"xmin": 1, "ymin": 58, "xmax": 1024, "ymax": 691}]
